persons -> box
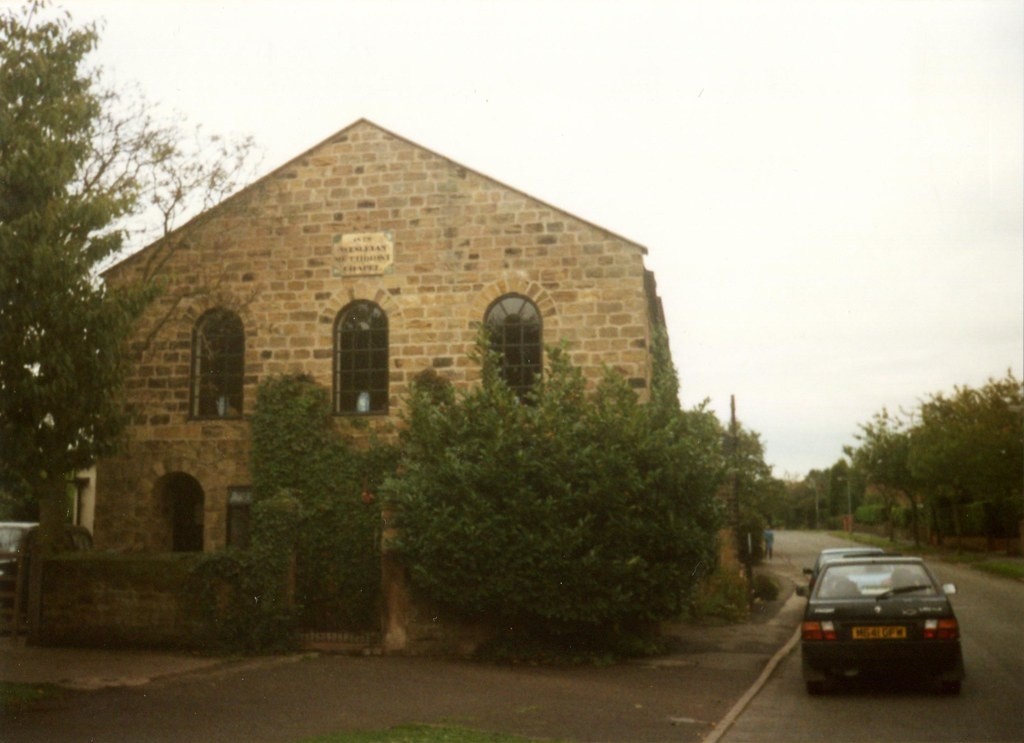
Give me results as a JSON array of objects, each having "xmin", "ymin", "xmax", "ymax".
[{"xmin": 764, "ymin": 530, "xmax": 773, "ymax": 561}]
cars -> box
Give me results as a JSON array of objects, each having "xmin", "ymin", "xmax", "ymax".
[
  {"xmin": 796, "ymin": 555, "xmax": 966, "ymax": 691},
  {"xmin": 802, "ymin": 546, "xmax": 896, "ymax": 599}
]
